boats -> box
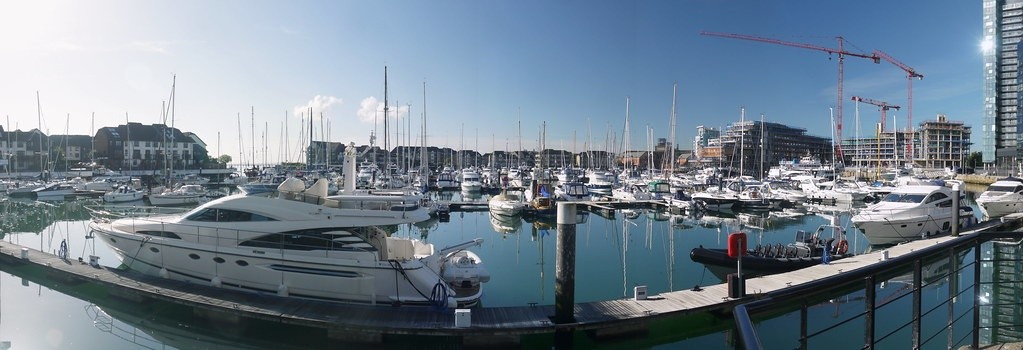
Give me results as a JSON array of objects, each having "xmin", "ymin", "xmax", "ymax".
[
  {"xmin": 89, "ymin": 192, "xmax": 493, "ymax": 317},
  {"xmin": 975, "ymin": 176, "xmax": 1023, "ymax": 220},
  {"xmin": 851, "ymin": 184, "xmax": 975, "ymax": 246},
  {"xmin": 688, "ymin": 225, "xmax": 853, "ymax": 282}
]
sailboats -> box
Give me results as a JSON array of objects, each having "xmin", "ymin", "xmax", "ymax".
[{"xmin": 0, "ymin": 65, "xmax": 964, "ymax": 220}]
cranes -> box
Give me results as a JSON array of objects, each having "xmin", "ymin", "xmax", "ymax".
[
  {"xmin": 851, "ymin": 95, "xmax": 902, "ymax": 133},
  {"xmin": 873, "ymin": 48, "xmax": 925, "ymax": 131},
  {"xmin": 698, "ymin": 30, "xmax": 880, "ymax": 158}
]
